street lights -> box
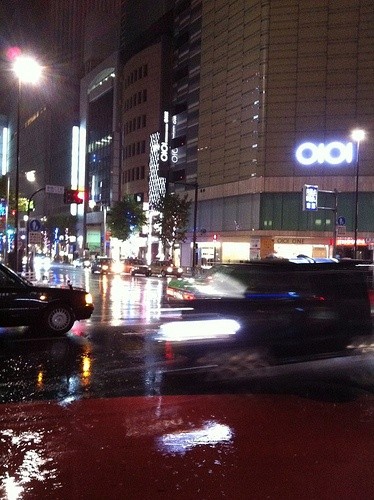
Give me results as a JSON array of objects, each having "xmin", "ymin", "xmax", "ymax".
[
  {"xmin": 353, "ymin": 128, "xmax": 364, "ymax": 259},
  {"xmin": 90, "ymin": 199, "xmax": 107, "ymax": 257},
  {"xmin": 173, "ymin": 181, "xmax": 199, "ymax": 277}
]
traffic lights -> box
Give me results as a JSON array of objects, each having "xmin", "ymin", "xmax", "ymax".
[{"xmin": 64, "ymin": 189, "xmax": 83, "ymax": 204}]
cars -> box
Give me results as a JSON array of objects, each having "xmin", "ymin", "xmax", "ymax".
[
  {"xmin": 0, "ymin": 263, "xmax": 94, "ymax": 335},
  {"xmin": 149, "ymin": 260, "xmax": 183, "ymax": 278},
  {"xmin": 73, "ymin": 257, "xmax": 119, "ymax": 275},
  {"xmin": 118, "ymin": 258, "xmax": 151, "ymax": 276}
]
